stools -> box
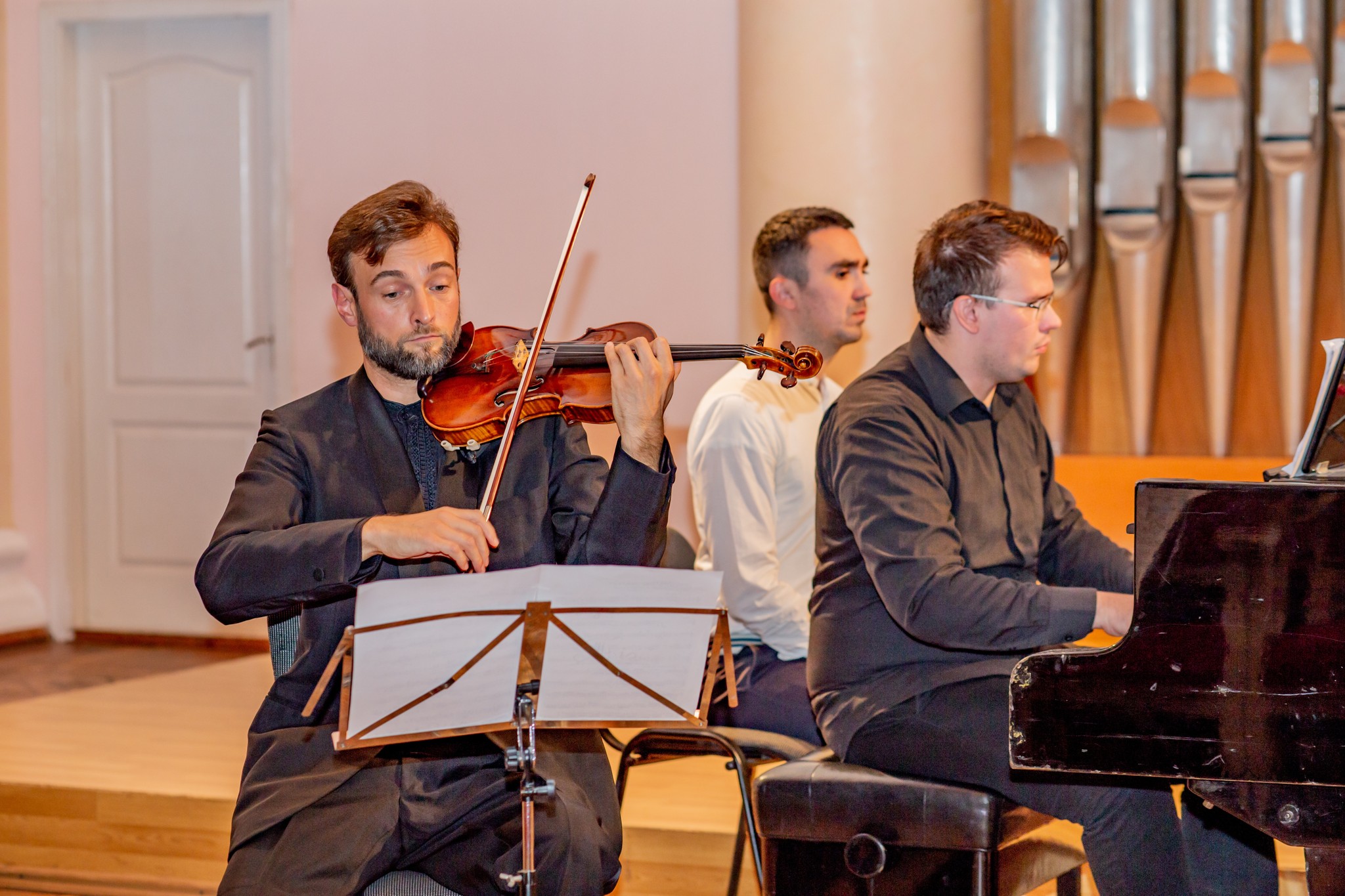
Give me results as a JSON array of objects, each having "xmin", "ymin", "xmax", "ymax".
[{"xmin": 747, "ymin": 745, "xmax": 1088, "ymax": 896}]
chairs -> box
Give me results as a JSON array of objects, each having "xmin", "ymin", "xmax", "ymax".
[{"xmin": 598, "ymin": 526, "xmax": 817, "ymax": 896}]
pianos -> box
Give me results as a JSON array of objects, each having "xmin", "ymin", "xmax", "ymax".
[{"xmin": 1006, "ymin": 469, "xmax": 1345, "ymax": 896}]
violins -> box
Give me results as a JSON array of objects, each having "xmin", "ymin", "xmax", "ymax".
[{"xmin": 417, "ymin": 321, "xmax": 824, "ymax": 450}]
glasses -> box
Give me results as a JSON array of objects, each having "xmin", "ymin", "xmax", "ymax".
[{"xmin": 945, "ymin": 294, "xmax": 1052, "ymax": 315}]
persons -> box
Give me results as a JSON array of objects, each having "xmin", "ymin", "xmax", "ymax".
[
  {"xmin": 685, "ymin": 197, "xmax": 1279, "ymax": 896},
  {"xmin": 194, "ymin": 182, "xmax": 676, "ymax": 896}
]
